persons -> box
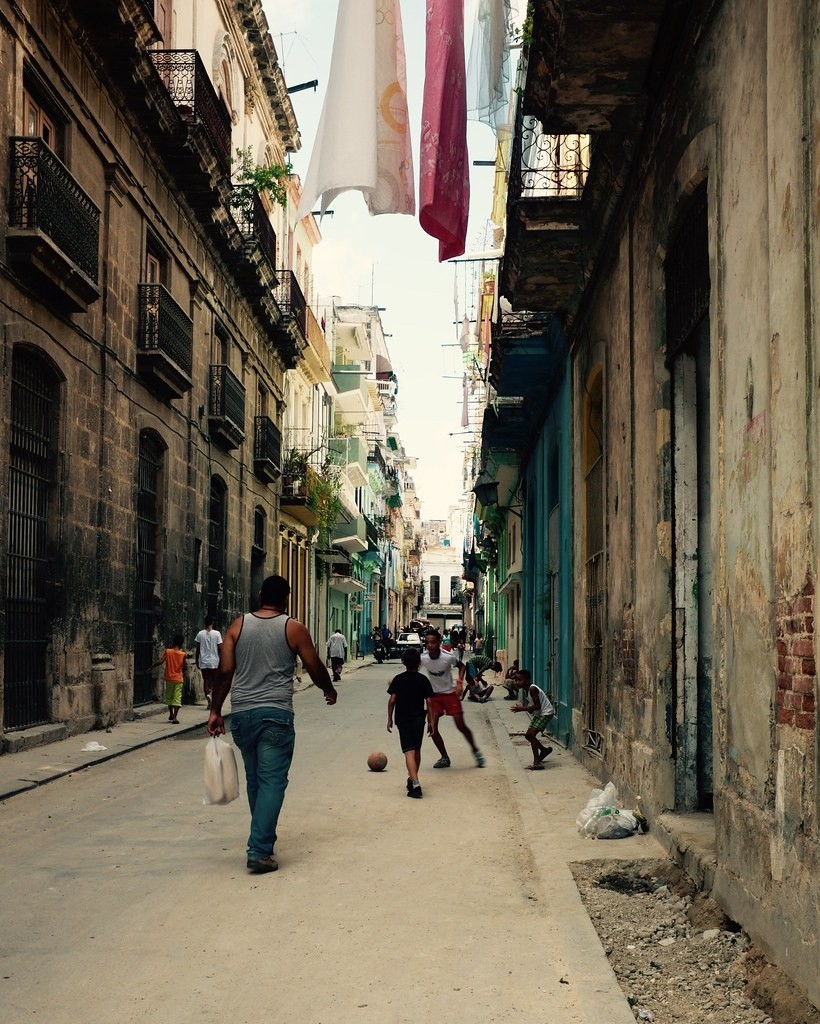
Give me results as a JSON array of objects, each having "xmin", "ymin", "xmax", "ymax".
[
  {"xmin": 435, "ymin": 625, "xmax": 476, "ymax": 651},
  {"xmin": 458, "ymin": 639, "xmax": 465, "ymax": 662},
  {"xmin": 195, "ymin": 616, "xmax": 223, "ymax": 710},
  {"xmin": 465, "ymin": 655, "xmax": 503, "ymax": 696},
  {"xmin": 325, "ymin": 628, "xmax": 348, "ymax": 683},
  {"xmin": 502, "ymin": 659, "xmax": 519, "ymax": 701},
  {"xmin": 459, "ymin": 674, "xmax": 494, "ymax": 704},
  {"xmin": 207, "ymin": 576, "xmax": 337, "ymax": 874},
  {"xmin": 388, "ymin": 628, "xmax": 486, "ymax": 767},
  {"xmin": 371, "ymin": 623, "xmax": 393, "ymax": 656},
  {"xmin": 474, "ymin": 632, "xmax": 485, "ymax": 655},
  {"xmin": 386, "ymin": 648, "xmax": 435, "ymax": 799},
  {"xmin": 150, "ymin": 634, "xmax": 188, "ymax": 724},
  {"xmin": 510, "ymin": 670, "xmax": 555, "ymax": 771},
  {"xmin": 440, "ymin": 628, "xmax": 452, "ymax": 652}
]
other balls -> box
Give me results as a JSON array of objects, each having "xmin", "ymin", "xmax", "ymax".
[{"xmin": 368, "ymin": 751, "xmax": 388, "ymax": 771}]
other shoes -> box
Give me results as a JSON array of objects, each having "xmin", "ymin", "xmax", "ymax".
[
  {"xmin": 333, "ymin": 671, "xmax": 341, "ymax": 682},
  {"xmin": 247, "ymin": 855, "xmax": 278, "ymax": 872},
  {"xmin": 504, "ymin": 695, "xmax": 517, "ymax": 700},
  {"xmin": 207, "ymin": 695, "xmax": 212, "ymax": 709},
  {"xmin": 406, "ymin": 778, "xmax": 422, "ymax": 797},
  {"xmin": 474, "ymin": 749, "xmax": 484, "ymax": 767},
  {"xmin": 433, "ymin": 757, "xmax": 451, "ymax": 769},
  {"xmin": 479, "ymin": 698, "xmax": 486, "ymax": 703},
  {"xmin": 168, "ymin": 714, "xmax": 179, "ymax": 724}
]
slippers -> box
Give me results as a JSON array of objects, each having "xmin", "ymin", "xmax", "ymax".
[
  {"xmin": 538, "ymin": 746, "xmax": 553, "ymax": 761},
  {"xmin": 525, "ymin": 762, "xmax": 544, "ymax": 770}
]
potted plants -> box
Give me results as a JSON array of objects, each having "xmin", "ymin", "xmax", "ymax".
[{"xmin": 283, "ymin": 448, "xmax": 331, "ymax": 516}]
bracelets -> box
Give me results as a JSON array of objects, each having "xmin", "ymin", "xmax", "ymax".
[{"xmin": 456, "ymin": 679, "xmax": 463, "ymax": 684}]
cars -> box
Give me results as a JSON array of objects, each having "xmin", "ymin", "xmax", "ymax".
[{"xmin": 396, "ymin": 633, "xmax": 424, "ymax": 652}]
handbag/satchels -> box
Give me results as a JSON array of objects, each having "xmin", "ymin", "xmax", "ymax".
[{"xmin": 202, "ymin": 727, "xmax": 240, "ymax": 807}]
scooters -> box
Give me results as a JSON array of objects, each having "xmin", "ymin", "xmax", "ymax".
[{"xmin": 372, "ymin": 641, "xmax": 390, "ymax": 664}]
